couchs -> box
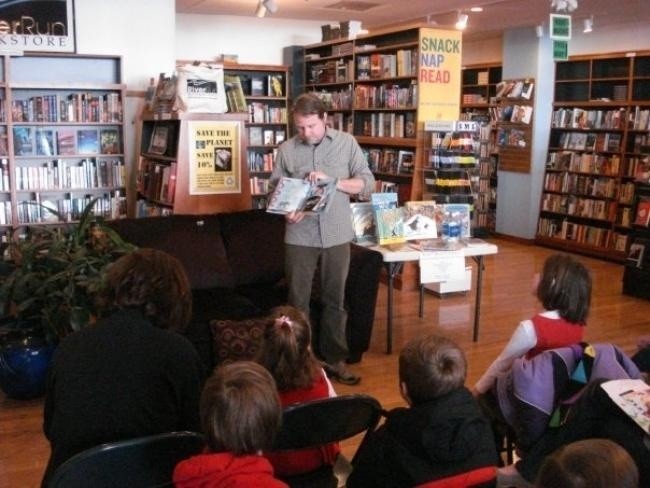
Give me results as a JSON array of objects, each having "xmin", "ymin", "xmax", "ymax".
[{"xmin": 92, "ymin": 213, "xmax": 383, "ymax": 364}]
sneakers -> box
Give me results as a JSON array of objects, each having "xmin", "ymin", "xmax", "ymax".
[{"xmin": 323, "ymin": 359, "xmax": 361, "ymax": 385}]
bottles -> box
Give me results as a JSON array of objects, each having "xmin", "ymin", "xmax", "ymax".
[{"xmin": 442, "ymin": 211, "xmax": 462, "ymax": 244}]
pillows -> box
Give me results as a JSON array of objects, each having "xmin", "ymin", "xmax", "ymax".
[{"xmin": 208, "ymin": 316, "xmax": 274, "ymax": 365}]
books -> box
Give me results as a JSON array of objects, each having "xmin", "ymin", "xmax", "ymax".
[
  {"xmin": 599, "ymin": 375, "xmax": 649, "ymax": 435},
  {"xmin": 531, "ymin": 96, "xmax": 650, "ymax": 267},
  {"xmin": 136, "ymin": 62, "xmax": 223, "ymax": 225},
  {"xmin": 224, "ymin": 37, "xmax": 539, "ymax": 233},
  {"xmin": 0, "ymin": 91, "xmax": 122, "ymax": 223},
  {"xmin": 349, "ymin": 231, "xmax": 474, "ymax": 248}
]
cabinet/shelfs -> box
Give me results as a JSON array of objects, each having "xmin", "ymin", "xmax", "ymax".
[
  {"xmin": 128, "ymin": 59, "xmax": 290, "ymax": 218},
  {"xmin": 0, "ymin": 51, "xmax": 130, "ymax": 244}
]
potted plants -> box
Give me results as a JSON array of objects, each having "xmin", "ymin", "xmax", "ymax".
[{"xmin": 0, "ymin": 196, "xmax": 138, "ymax": 400}]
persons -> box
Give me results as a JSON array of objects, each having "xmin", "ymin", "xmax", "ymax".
[
  {"xmin": 171, "ymin": 357, "xmax": 294, "ymax": 488},
  {"xmin": 467, "ymin": 252, "xmax": 594, "ymax": 428},
  {"xmin": 343, "ymin": 331, "xmax": 500, "ymax": 488},
  {"xmin": 39, "ymin": 245, "xmax": 197, "ymax": 486},
  {"xmin": 246, "ymin": 304, "xmax": 344, "ymax": 487},
  {"xmin": 524, "ymin": 436, "xmax": 643, "ymax": 488},
  {"xmin": 265, "ymin": 88, "xmax": 375, "ymax": 388}
]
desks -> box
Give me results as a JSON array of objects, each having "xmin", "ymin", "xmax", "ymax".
[{"xmin": 350, "ymin": 235, "xmax": 498, "ymax": 354}]
